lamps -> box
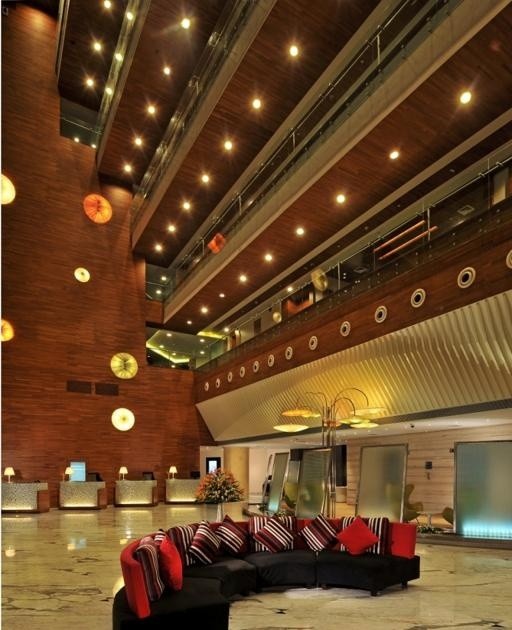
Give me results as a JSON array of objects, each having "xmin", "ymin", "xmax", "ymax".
[
  {"xmin": 118, "ymin": 466, "xmax": 129, "ymax": 481},
  {"xmin": 168, "ymin": 465, "xmax": 177, "ymax": 480},
  {"xmin": 64, "ymin": 466, "xmax": 73, "ymax": 483},
  {"xmin": 3, "ymin": 466, "xmax": 15, "ymax": 484}
]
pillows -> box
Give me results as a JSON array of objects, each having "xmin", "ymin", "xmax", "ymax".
[{"xmin": 132, "ymin": 513, "xmax": 390, "ymax": 605}]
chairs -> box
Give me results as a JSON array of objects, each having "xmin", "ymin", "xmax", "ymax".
[{"xmin": 403, "ymin": 484, "xmax": 432, "ymax": 530}]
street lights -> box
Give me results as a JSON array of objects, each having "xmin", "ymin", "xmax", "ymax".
[{"xmin": 274, "ymin": 388, "xmax": 387, "ymax": 518}]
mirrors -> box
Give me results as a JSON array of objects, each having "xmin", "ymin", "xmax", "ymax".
[
  {"xmin": 453, "ymin": 439, "xmax": 512, "ymax": 542},
  {"xmin": 266, "ymin": 452, "xmax": 290, "ymax": 516},
  {"xmin": 354, "ymin": 443, "xmax": 408, "ymax": 523},
  {"xmin": 206, "ymin": 457, "xmax": 222, "ymax": 475},
  {"xmin": 295, "ymin": 447, "xmax": 334, "ymax": 519}
]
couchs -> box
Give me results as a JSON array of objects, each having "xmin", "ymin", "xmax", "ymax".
[{"xmin": 111, "ymin": 519, "xmax": 421, "ymax": 630}]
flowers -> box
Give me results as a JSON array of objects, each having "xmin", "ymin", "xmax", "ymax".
[{"xmin": 192, "ymin": 467, "xmax": 244, "ymax": 504}]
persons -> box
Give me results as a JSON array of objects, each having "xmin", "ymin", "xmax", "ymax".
[{"xmin": 262, "ymin": 475, "xmax": 272, "ymax": 496}]
